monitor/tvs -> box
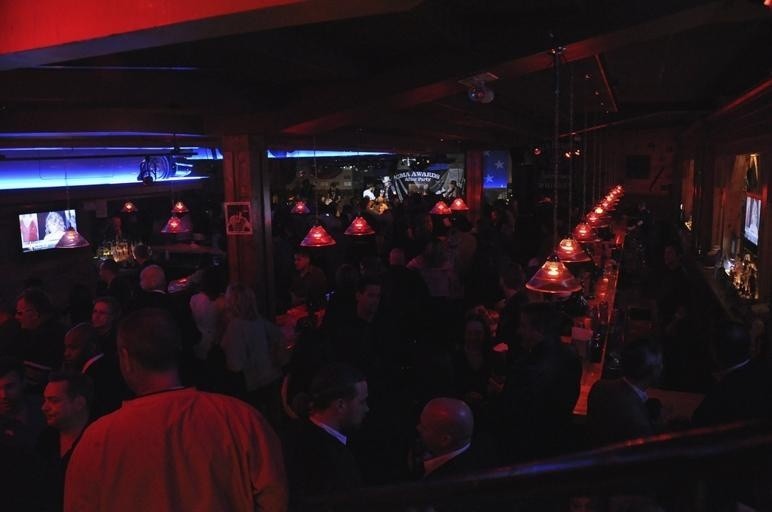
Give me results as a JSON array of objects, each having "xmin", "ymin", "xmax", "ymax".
[
  {"xmin": 16, "ymin": 207, "xmax": 78, "ymax": 257},
  {"xmin": 742, "ymin": 193, "xmax": 762, "ymax": 255}
]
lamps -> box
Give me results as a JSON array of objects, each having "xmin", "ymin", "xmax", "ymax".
[
  {"xmin": 525, "ymin": 51, "xmax": 583, "ymax": 295},
  {"xmin": 429, "ymin": 201, "xmax": 452, "ymax": 215},
  {"xmin": 299, "ymin": 133, "xmax": 336, "ymax": 248},
  {"xmin": 291, "ymin": 200, "xmax": 310, "ymax": 214},
  {"xmin": 572, "ymin": 75, "xmax": 643, "ymax": 244},
  {"xmin": 450, "ymin": 168, "xmax": 469, "ymax": 211},
  {"xmin": 120, "ymin": 184, "xmax": 192, "ymax": 234},
  {"xmin": 54, "ymin": 152, "xmax": 90, "ymax": 249},
  {"xmin": 552, "ymin": 64, "xmax": 591, "ymax": 263},
  {"xmin": 343, "ymin": 216, "xmax": 375, "ymax": 236}
]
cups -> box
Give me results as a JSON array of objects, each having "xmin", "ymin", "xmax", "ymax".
[{"xmin": 97, "ymin": 237, "xmax": 144, "ymax": 260}]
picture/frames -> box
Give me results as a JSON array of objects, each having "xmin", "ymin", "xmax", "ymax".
[{"xmin": 224, "ymin": 201, "xmax": 253, "ymax": 236}]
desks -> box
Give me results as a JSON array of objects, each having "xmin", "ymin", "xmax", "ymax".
[{"xmin": 567, "ymin": 214, "xmax": 629, "ymax": 415}]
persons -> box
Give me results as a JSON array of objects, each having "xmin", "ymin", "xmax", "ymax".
[{"xmin": 2, "ymin": 192, "xmax": 771, "ymax": 512}]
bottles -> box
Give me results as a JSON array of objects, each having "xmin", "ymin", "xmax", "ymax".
[
  {"xmin": 591, "ymin": 301, "xmax": 608, "ymax": 326},
  {"xmin": 588, "ymin": 329, "xmax": 602, "ymax": 363}
]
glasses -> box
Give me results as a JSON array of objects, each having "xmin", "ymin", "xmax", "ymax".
[{"xmin": 14, "ymin": 307, "xmax": 35, "ymax": 316}]
